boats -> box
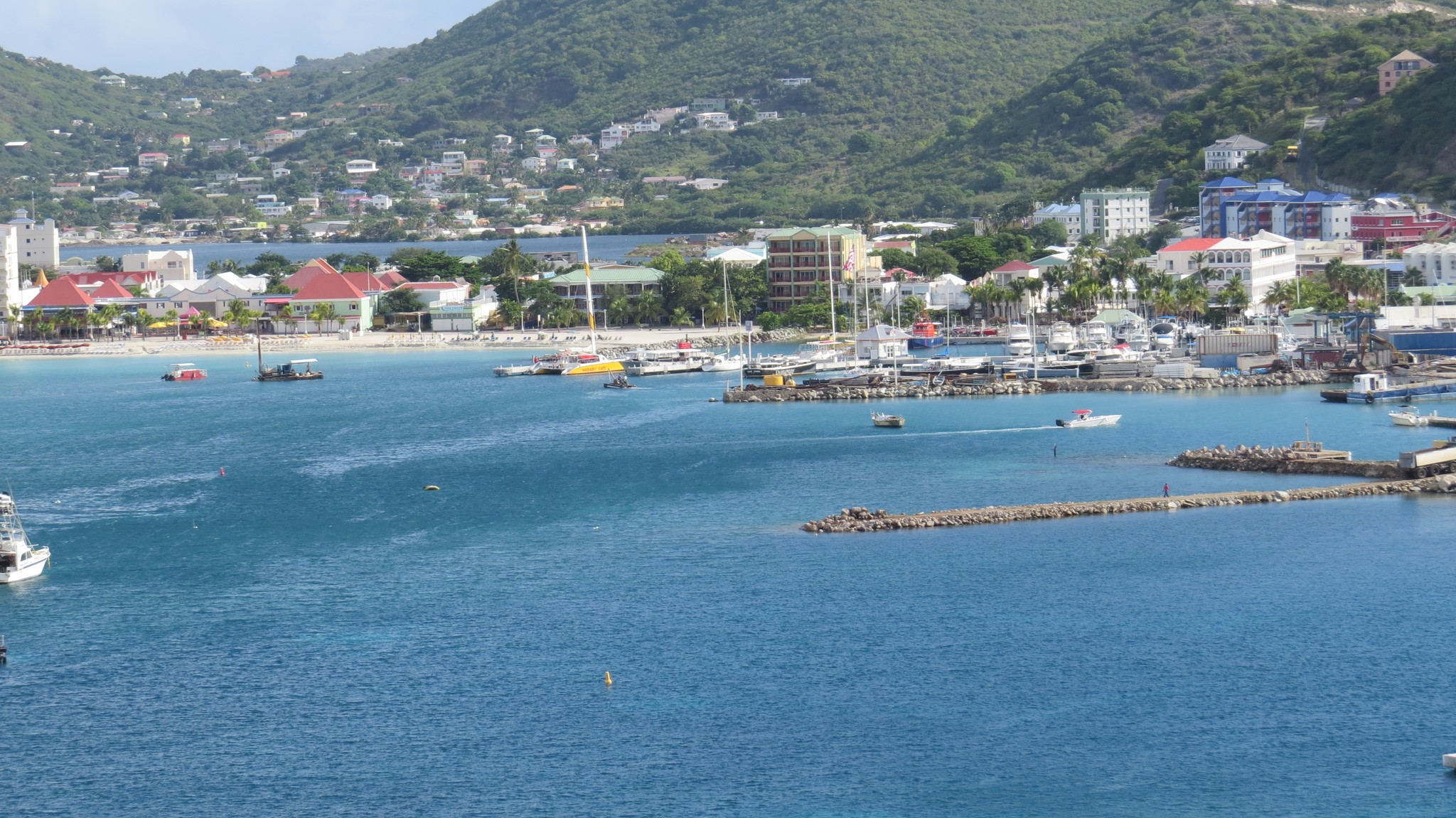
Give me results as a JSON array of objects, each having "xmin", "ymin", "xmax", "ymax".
[
  {"xmin": 162, "ymin": 363, "xmax": 208, "ymax": 381},
  {"xmin": 744, "ymin": 354, "xmax": 817, "ymax": 378},
  {"xmin": 603, "ymin": 368, "xmax": 637, "ymax": 389},
  {"xmin": 1, "ymin": 492, "xmax": 50, "ymax": 584},
  {"xmin": 899, "ymin": 356, "xmax": 1096, "ymax": 379},
  {"xmin": 1389, "ymin": 408, "xmax": 1429, "ymax": 428},
  {"xmin": 1055, "ymin": 410, "xmax": 1123, "ymax": 429},
  {"xmin": 1289, "ymin": 415, "xmax": 1352, "ymax": 461},
  {"xmin": 0, "ymin": 336, "xmax": 14, "ymax": 349},
  {"xmin": 492, "ymin": 225, "xmax": 716, "ymax": 377},
  {"xmin": 869, "ymin": 409, "xmax": 905, "ymax": 426},
  {"xmin": 255, "ymin": 317, "xmax": 324, "ymax": 382},
  {"xmin": 1317, "ymin": 369, "xmax": 1456, "ymax": 405},
  {"xmin": 904, "ymin": 297, "xmax": 1297, "ymax": 360}
]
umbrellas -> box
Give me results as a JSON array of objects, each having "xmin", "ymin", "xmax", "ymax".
[
  {"xmin": 149, "ymin": 323, "xmax": 166, "ymax": 328},
  {"xmin": 207, "ymin": 319, "xmax": 228, "ymax": 327}
]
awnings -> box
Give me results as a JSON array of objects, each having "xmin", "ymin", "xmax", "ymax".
[{"xmin": 264, "ymin": 298, "xmax": 291, "ymax": 304}]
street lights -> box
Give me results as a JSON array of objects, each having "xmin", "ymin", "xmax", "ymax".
[
  {"xmin": 739, "ymin": 208, "xmax": 743, "ymax": 218},
  {"xmin": 690, "ymin": 208, "xmax": 694, "ymax": 217},
  {"xmin": 643, "ymin": 209, "xmax": 648, "ymax": 218},
  {"xmin": 789, "ymin": 207, "xmax": 793, "ymax": 219},
  {"xmin": 890, "ymin": 207, "xmax": 895, "ymax": 214},
  {"xmin": 841, "ymin": 207, "xmax": 844, "ymax": 220},
  {"xmin": 942, "ymin": 205, "xmax": 946, "ymax": 219}
]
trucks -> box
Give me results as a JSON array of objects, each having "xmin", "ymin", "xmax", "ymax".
[{"xmin": 1398, "ymin": 435, "xmax": 1456, "ymax": 478}]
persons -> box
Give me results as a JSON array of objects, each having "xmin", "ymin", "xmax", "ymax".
[
  {"xmin": 456, "ymin": 324, "xmax": 459, "ymax": 332},
  {"xmin": 1163, "ymin": 483, "xmax": 1169, "ymax": 497}
]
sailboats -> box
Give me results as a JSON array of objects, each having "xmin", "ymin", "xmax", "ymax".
[
  {"xmin": 701, "ymin": 258, "xmax": 748, "ymax": 372},
  {"xmin": 796, "ymin": 223, "xmax": 912, "ymax": 362}
]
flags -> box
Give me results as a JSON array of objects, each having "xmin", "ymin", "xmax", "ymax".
[{"xmin": 842, "ymin": 244, "xmax": 855, "ymax": 273}]
